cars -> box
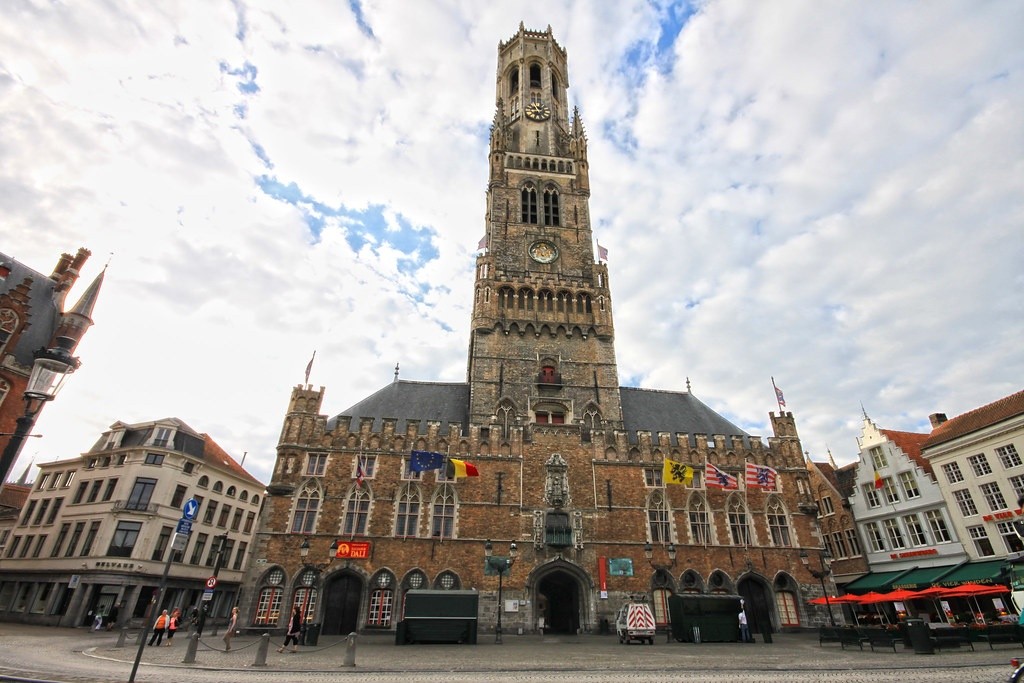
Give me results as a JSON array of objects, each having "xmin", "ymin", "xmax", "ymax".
[{"xmin": 615, "ymin": 602, "xmax": 656, "ymax": 645}]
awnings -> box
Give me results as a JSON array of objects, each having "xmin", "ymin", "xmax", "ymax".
[{"xmin": 843, "ymin": 559, "xmax": 1006, "ymax": 593}]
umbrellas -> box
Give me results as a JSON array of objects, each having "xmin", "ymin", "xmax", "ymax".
[{"xmin": 808, "ymin": 582, "xmax": 1011, "ymax": 628}]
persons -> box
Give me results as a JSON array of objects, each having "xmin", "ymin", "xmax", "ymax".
[
  {"xmin": 222, "ymin": 607, "xmax": 241, "ymax": 651},
  {"xmin": 147, "ymin": 607, "xmax": 182, "ymax": 646},
  {"xmin": 190, "ymin": 607, "xmax": 198, "ymax": 624},
  {"xmin": 106, "ymin": 604, "xmax": 119, "ymax": 631},
  {"xmin": 738, "ymin": 599, "xmax": 750, "ymax": 641},
  {"xmin": 276, "ymin": 607, "xmax": 301, "ymax": 653},
  {"xmin": 88, "ymin": 605, "xmax": 105, "ymax": 633}
]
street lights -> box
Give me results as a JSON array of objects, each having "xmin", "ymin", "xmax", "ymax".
[
  {"xmin": 644, "ymin": 542, "xmax": 677, "ymax": 643},
  {"xmin": 484, "ymin": 538, "xmax": 518, "ymax": 644},
  {"xmin": 800, "ymin": 549, "xmax": 835, "ymax": 626},
  {"xmin": 297, "ymin": 536, "xmax": 339, "ymax": 646},
  {"xmin": 0, "ymin": 335, "xmax": 83, "ymax": 486}
]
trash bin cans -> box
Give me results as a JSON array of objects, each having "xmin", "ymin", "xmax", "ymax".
[
  {"xmin": 306, "ymin": 619, "xmax": 320, "ymax": 646},
  {"xmin": 907, "ymin": 618, "xmax": 935, "ymax": 655}
]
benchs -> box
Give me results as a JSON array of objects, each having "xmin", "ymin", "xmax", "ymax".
[{"xmin": 818, "ymin": 624, "xmax": 1024, "ymax": 653}]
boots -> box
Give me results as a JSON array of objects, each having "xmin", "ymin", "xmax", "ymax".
[
  {"xmin": 291, "ymin": 645, "xmax": 298, "ymax": 653},
  {"xmin": 166, "ymin": 637, "xmax": 173, "ymax": 646},
  {"xmin": 276, "ymin": 645, "xmax": 286, "ymax": 653}
]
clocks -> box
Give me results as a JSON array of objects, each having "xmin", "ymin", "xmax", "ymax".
[{"xmin": 525, "ymin": 101, "xmax": 551, "ymax": 122}]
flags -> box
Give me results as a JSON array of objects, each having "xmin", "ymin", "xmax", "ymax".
[
  {"xmin": 597, "ymin": 245, "xmax": 608, "ymax": 260},
  {"xmin": 356, "ymin": 455, "xmax": 365, "ymax": 489},
  {"xmin": 662, "ymin": 457, "xmax": 693, "ymax": 486},
  {"xmin": 775, "ymin": 386, "xmax": 785, "ymax": 407},
  {"xmin": 873, "ymin": 465, "xmax": 884, "ymax": 488},
  {"xmin": 305, "ymin": 354, "xmax": 314, "ymax": 383},
  {"xmin": 705, "ymin": 461, "xmax": 738, "ymax": 490},
  {"xmin": 446, "ymin": 457, "xmax": 479, "ymax": 478},
  {"xmin": 746, "ymin": 462, "xmax": 777, "ymax": 489},
  {"xmin": 477, "ymin": 235, "xmax": 486, "ymax": 250},
  {"xmin": 409, "ymin": 450, "xmax": 444, "ymax": 472}
]
